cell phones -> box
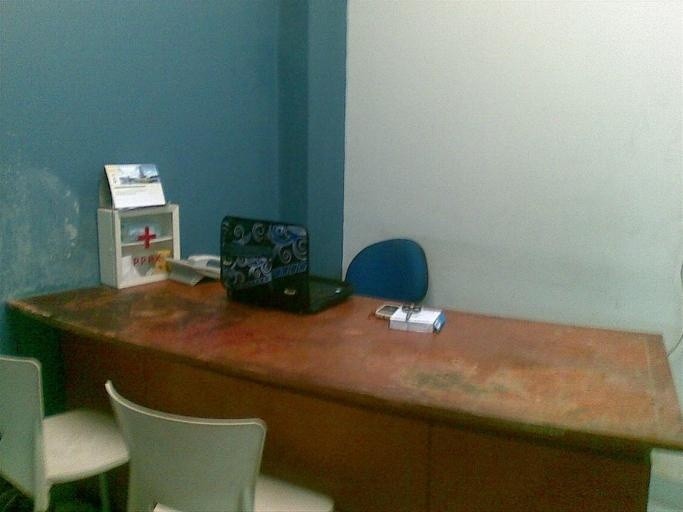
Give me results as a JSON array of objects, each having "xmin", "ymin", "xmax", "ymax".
[{"xmin": 374, "ymin": 304, "xmax": 400, "ymax": 322}]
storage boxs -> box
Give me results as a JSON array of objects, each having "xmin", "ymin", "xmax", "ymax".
[{"xmin": 97, "ymin": 204, "xmax": 182, "ymax": 290}]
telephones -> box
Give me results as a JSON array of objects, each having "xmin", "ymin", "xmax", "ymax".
[{"xmin": 166, "ymin": 253, "xmax": 221, "ymax": 287}]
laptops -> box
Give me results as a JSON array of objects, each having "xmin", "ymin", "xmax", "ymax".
[{"xmin": 219, "ymin": 218, "xmax": 353, "ymax": 316}]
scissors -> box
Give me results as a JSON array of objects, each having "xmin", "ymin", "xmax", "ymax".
[{"xmin": 402, "ymin": 305, "xmax": 421, "ymax": 321}]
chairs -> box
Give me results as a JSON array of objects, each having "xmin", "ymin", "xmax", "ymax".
[
  {"xmin": 99, "ymin": 379, "xmax": 336, "ymax": 512},
  {"xmin": 0, "ymin": 353, "xmax": 129, "ymax": 512},
  {"xmin": 343, "ymin": 237, "xmax": 428, "ymax": 306}
]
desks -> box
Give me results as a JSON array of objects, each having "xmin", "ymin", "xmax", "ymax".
[{"xmin": 4, "ymin": 275, "xmax": 682, "ymax": 510}]
